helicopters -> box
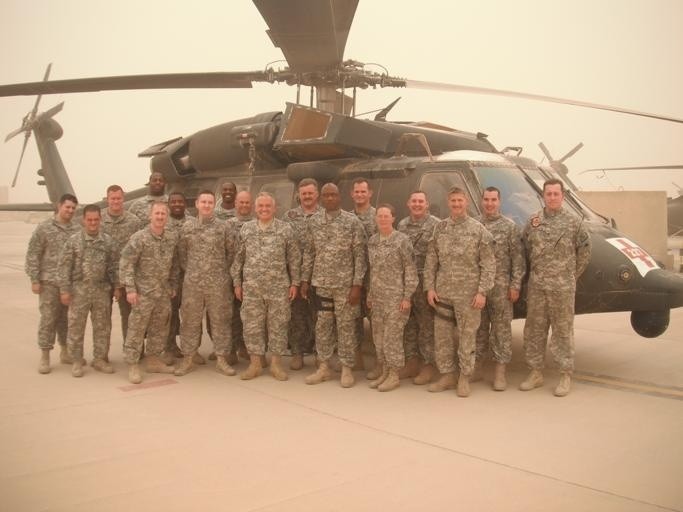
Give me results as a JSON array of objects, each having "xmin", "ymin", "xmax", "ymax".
[{"xmin": 0, "ymin": 0, "xmax": 683, "ymax": 339}]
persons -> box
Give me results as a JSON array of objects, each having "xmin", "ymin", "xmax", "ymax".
[
  {"xmin": 280, "ymin": 177, "xmax": 441, "ymax": 391},
  {"xmin": 422, "ymin": 185, "xmax": 496, "ymax": 396},
  {"xmin": 120, "ymin": 172, "xmax": 302, "ymax": 383},
  {"xmin": 98, "ymin": 184, "xmax": 142, "ymax": 363},
  {"xmin": 56, "ymin": 203, "xmax": 123, "ymax": 377},
  {"xmin": 25, "ymin": 193, "xmax": 87, "ymax": 373},
  {"xmin": 519, "ymin": 179, "xmax": 592, "ymax": 396},
  {"xmin": 468, "ymin": 183, "xmax": 526, "ymax": 391}
]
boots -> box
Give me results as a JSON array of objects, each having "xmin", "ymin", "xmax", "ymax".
[
  {"xmin": 492, "ymin": 362, "xmax": 507, "ymax": 391},
  {"xmin": 365, "ymin": 364, "xmax": 400, "ymax": 392},
  {"xmin": 174, "ymin": 350, "xmax": 240, "ymax": 378},
  {"xmin": 290, "ymin": 354, "xmax": 356, "ymax": 387},
  {"xmin": 239, "ymin": 352, "xmax": 303, "ymax": 381},
  {"xmin": 517, "ymin": 369, "xmax": 570, "ymax": 398},
  {"xmin": 37, "ymin": 349, "xmax": 116, "ymax": 377},
  {"xmin": 425, "ymin": 370, "xmax": 471, "ymax": 398},
  {"xmin": 124, "ymin": 344, "xmax": 182, "ymax": 386},
  {"xmin": 399, "ymin": 359, "xmax": 433, "ymax": 386}
]
